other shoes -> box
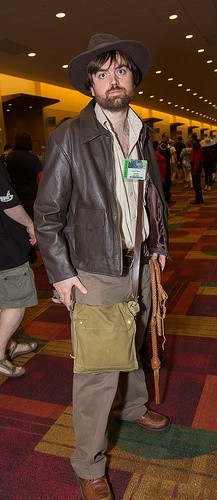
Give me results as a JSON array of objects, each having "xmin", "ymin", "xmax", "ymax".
[
  {"xmin": 204, "ymin": 188, "xmax": 207, "ymax": 191},
  {"xmin": 207, "ymin": 189, "xmax": 211, "ymax": 192},
  {"xmin": 175, "ymin": 174, "xmax": 178, "ymax": 179},
  {"xmin": 171, "ymin": 179, "xmax": 175, "ymax": 186}
]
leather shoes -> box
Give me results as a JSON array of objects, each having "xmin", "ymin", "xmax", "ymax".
[
  {"xmin": 130, "ymin": 409, "xmax": 170, "ymax": 431},
  {"xmin": 169, "ymin": 199, "xmax": 176, "ymax": 203},
  {"xmin": 77, "ymin": 475, "xmax": 114, "ymax": 500},
  {"xmin": 190, "ymin": 198, "xmax": 204, "ymax": 204}
]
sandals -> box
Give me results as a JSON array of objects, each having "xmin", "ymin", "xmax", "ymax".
[
  {"xmin": 6, "ymin": 339, "xmax": 37, "ymax": 361},
  {"xmin": 0, "ymin": 357, "xmax": 26, "ymax": 377}
]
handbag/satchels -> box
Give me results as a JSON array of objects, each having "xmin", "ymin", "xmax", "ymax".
[{"xmin": 70, "ymin": 298, "xmax": 139, "ymax": 375}]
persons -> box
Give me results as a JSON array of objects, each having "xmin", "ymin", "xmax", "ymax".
[
  {"xmin": 158, "ymin": 132, "xmax": 176, "ymax": 203},
  {"xmin": 0, "ymin": 163, "xmax": 39, "ymax": 379},
  {"xmin": 188, "ymin": 134, "xmax": 204, "ymax": 204},
  {"xmin": 167, "ymin": 135, "xmax": 217, "ymax": 191},
  {"xmin": 0, "ymin": 131, "xmax": 62, "ymax": 304},
  {"xmin": 152, "ymin": 141, "xmax": 166, "ymax": 193},
  {"xmin": 33, "ymin": 33, "xmax": 172, "ymax": 500}
]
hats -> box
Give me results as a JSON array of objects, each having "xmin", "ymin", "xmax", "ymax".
[
  {"xmin": 199, "ymin": 137, "xmax": 216, "ymax": 147},
  {"xmin": 67, "ymin": 33, "xmax": 151, "ymax": 98}
]
